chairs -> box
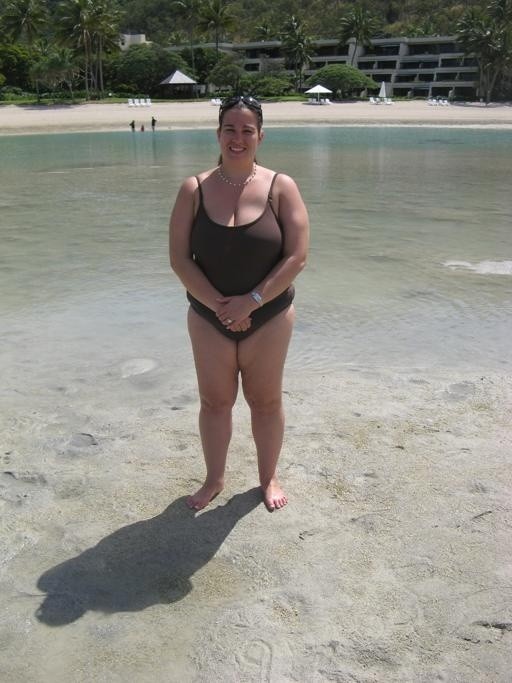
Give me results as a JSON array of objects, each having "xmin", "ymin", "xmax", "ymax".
[
  {"xmin": 127, "ymin": 98, "xmax": 152, "ymax": 108},
  {"xmin": 302, "ymin": 97, "xmax": 451, "ymax": 107},
  {"xmin": 210, "ymin": 98, "xmax": 222, "ymax": 106}
]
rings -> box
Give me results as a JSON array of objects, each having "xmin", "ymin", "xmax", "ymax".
[{"xmin": 227, "ymin": 318, "xmax": 232, "ymax": 323}]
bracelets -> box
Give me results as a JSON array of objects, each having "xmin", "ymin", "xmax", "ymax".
[{"xmin": 250, "ymin": 291, "xmax": 263, "ymax": 307}]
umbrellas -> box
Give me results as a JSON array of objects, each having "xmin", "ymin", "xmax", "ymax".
[
  {"xmin": 378, "ymin": 81, "xmax": 386, "ymax": 102},
  {"xmin": 304, "ymin": 84, "xmax": 333, "ymax": 102}
]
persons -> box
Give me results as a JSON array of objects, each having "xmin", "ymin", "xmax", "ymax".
[
  {"xmin": 447, "ymin": 84, "xmax": 456, "ymax": 104},
  {"xmin": 165, "ymin": 94, "xmax": 315, "ymax": 513},
  {"xmin": 129, "ymin": 120, "xmax": 135, "ymax": 131},
  {"xmin": 151, "ymin": 115, "xmax": 157, "ymax": 132}
]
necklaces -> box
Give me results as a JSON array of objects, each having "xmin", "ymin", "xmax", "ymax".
[{"xmin": 217, "ymin": 162, "xmax": 257, "ymax": 186}]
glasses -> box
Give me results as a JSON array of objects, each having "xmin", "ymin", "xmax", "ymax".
[{"xmin": 218, "ymin": 94, "xmax": 263, "ymax": 125}]
